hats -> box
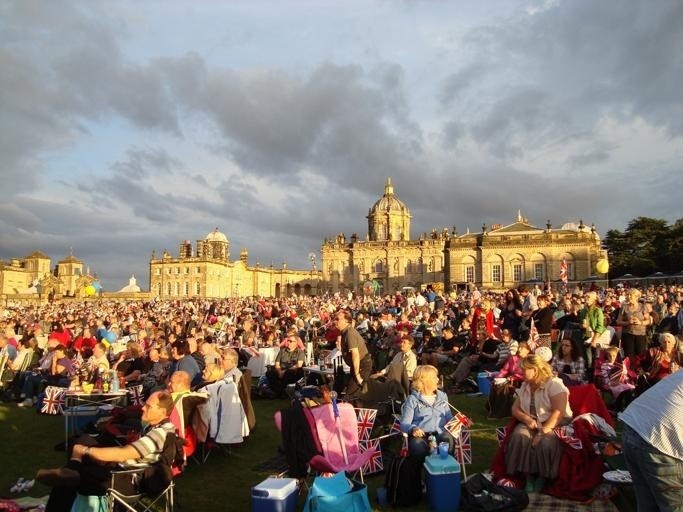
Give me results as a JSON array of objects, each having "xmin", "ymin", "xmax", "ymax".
[{"xmin": 534, "ymin": 347, "xmax": 553, "ymax": 363}]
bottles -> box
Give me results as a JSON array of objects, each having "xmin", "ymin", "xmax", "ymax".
[
  {"xmin": 110, "ymin": 378, "xmax": 113, "ymax": 392},
  {"xmin": 102, "ymin": 381, "xmax": 109, "ymax": 393},
  {"xmin": 113, "ymin": 372, "xmax": 119, "ymax": 392}
]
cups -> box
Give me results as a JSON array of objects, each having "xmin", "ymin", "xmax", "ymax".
[{"xmin": 439, "ymin": 442, "xmax": 450, "ymax": 459}]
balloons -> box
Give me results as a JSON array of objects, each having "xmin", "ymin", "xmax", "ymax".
[
  {"xmin": 595, "ymin": 259, "xmax": 611, "ymax": 275},
  {"xmin": 85, "ymin": 286, "xmax": 95, "ymax": 294},
  {"xmin": 92, "ymin": 281, "xmax": 101, "ymax": 291}
]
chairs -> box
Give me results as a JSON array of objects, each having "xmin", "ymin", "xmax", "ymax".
[
  {"xmin": 192, "ymin": 377, "xmax": 235, "ymax": 449},
  {"xmin": 235, "ymin": 367, "xmax": 254, "ymax": 439},
  {"xmin": 500, "ymin": 383, "xmax": 597, "ymax": 486},
  {"xmin": 384, "ymin": 399, "xmax": 472, "ymax": 511},
  {"xmin": 173, "ymin": 391, "xmax": 212, "ymax": 468},
  {"xmin": 79, "ymin": 428, "xmax": 179, "ymax": 512},
  {"xmin": 594, "ymin": 345, "xmax": 626, "ymax": 412}
]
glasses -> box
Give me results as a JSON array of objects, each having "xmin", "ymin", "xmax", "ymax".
[
  {"xmin": 333, "ymin": 318, "xmax": 344, "ymax": 322},
  {"xmin": 287, "ymin": 339, "xmax": 296, "ymax": 343},
  {"xmin": 221, "ymin": 355, "xmax": 231, "ymax": 360}
]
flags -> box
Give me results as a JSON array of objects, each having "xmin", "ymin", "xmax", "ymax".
[
  {"xmin": 389, "ymin": 419, "xmax": 403, "ymax": 440},
  {"xmin": 555, "ymin": 427, "xmax": 581, "ymax": 451},
  {"xmin": 451, "ymin": 430, "xmax": 471, "ymax": 464},
  {"xmin": 607, "ymin": 363, "xmax": 628, "ymax": 382},
  {"xmin": 128, "ymin": 383, "xmax": 145, "ymax": 402},
  {"xmin": 356, "ymin": 409, "xmax": 377, "ymax": 441},
  {"xmin": 496, "ymin": 428, "xmax": 507, "ymax": 445},
  {"xmin": 41, "ymin": 386, "xmax": 62, "ymax": 415},
  {"xmin": 357, "ymin": 440, "xmax": 383, "ymax": 475},
  {"xmin": 559, "ymin": 261, "xmax": 567, "ymax": 283},
  {"xmin": 443, "ymin": 412, "xmax": 470, "ymax": 438}
]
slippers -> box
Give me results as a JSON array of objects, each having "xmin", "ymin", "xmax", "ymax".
[{"xmin": 444, "ymin": 375, "xmax": 457, "ymax": 380}]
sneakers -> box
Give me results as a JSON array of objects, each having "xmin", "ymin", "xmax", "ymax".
[
  {"xmin": 36, "ymin": 468, "xmax": 79, "ymax": 485},
  {"xmin": 17, "ymin": 398, "xmax": 33, "ymax": 409}
]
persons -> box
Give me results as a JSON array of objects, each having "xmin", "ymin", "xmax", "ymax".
[
  {"xmin": 190, "ymin": 362, "xmax": 223, "ymax": 391},
  {"xmin": 373, "ymin": 334, "xmax": 417, "ymax": 382},
  {"xmin": 488, "ymin": 341, "xmax": 536, "ymax": 425},
  {"xmin": 0, "ymin": 296, "xmax": 169, "ymax": 388},
  {"xmin": 217, "ymin": 349, "xmax": 239, "ymax": 383},
  {"xmin": 85, "ymin": 341, "xmax": 109, "ymax": 370},
  {"xmin": 165, "ymin": 281, "xmax": 496, "ymax": 358},
  {"xmin": 496, "ymin": 281, "xmax": 613, "ymax": 347},
  {"xmin": 552, "ymin": 337, "xmax": 588, "ymax": 385},
  {"xmin": 18, "ymin": 344, "xmax": 73, "ymax": 408},
  {"xmin": 170, "ymin": 338, "xmax": 200, "ymax": 379},
  {"xmin": 483, "ymin": 328, "xmax": 518, "ymax": 371},
  {"xmin": 398, "ymin": 364, "xmax": 459, "ymax": 507},
  {"xmin": 34, "ymin": 390, "xmax": 183, "ymax": 512},
  {"xmin": 617, "ymin": 367, "xmax": 682, "ymax": 510},
  {"xmin": 333, "ymin": 311, "xmax": 372, "ymax": 402},
  {"xmin": 265, "ymin": 335, "xmax": 307, "ymax": 398},
  {"xmin": 506, "ymin": 354, "xmax": 574, "ymax": 493}
]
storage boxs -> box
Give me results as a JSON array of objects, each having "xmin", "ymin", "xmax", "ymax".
[
  {"xmin": 423, "ymin": 453, "xmax": 463, "ymax": 511},
  {"xmin": 251, "ymin": 476, "xmax": 299, "ymax": 511},
  {"xmin": 477, "ymin": 372, "xmax": 500, "ymax": 395}
]
